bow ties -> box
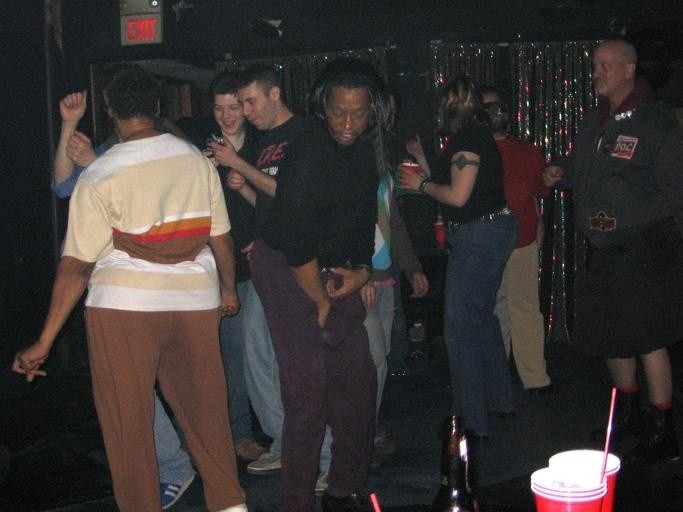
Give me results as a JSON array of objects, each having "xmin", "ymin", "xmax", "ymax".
[{"xmin": 159, "ymin": 469, "xmax": 196, "ymax": 509}]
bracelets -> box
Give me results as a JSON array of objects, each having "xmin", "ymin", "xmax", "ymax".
[
  {"xmin": 418, "ymin": 180, "xmax": 430, "ymax": 194},
  {"xmin": 355, "ymin": 266, "xmax": 372, "ymax": 281},
  {"xmin": 312, "ymin": 294, "xmax": 328, "ymax": 304}
]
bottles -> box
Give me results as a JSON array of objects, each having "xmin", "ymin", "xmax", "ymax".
[{"xmin": 430, "ymin": 415, "xmax": 479, "ymax": 512}]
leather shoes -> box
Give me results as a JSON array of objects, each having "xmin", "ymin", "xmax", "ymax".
[{"xmin": 528, "ymin": 382, "xmax": 560, "ymax": 395}]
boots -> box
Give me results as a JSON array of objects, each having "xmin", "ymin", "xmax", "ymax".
[
  {"xmin": 622, "ymin": 406, "xmax": 679, "ymax": 465},
  {"xmin": 596, "ymin": 391, "xmax": 643, "ymax": 445}
]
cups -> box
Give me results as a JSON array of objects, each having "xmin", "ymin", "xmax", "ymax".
[
  {"xmin": 531, "ymin": 449, "xmax": 621, "ymax": 512},
  {"xmin": 434, "ymin": 223, "xmax": 446, "ymax": 242},
  {"xmin": 400, "ymin": 162, "xmax": 419, "ymax": 186}
]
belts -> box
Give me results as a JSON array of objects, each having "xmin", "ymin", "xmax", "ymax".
[{"xmin": 443, "ymin": 205, "xmax": 511, "ymax": 229}]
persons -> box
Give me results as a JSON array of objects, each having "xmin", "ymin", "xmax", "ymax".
[
  {"xmin": 250, "ymin": 56, "xmax": 380, "ymax": 512},
  {"xmin": 394, "ymin": 71, "xmax": 520, "ymax": 452},
  {"xmin": 541, "ymin": 31, "xmax": 682, "ymax": 462},
  {"xmin": 361, "ymin": 169, "xmax": 430, "ymax": 472},
  {"xmin": 47, "ymin": 65, "xmax": 200, "ymax": 509},
  {"xmin": 188, "ymin": 68, "xmax": 269, "ymax": 461},
  {"xmin": 11, "ymin": 64, "xmax": 251, "ymax": 512},
  {"xmin": 300, "ymin": 57, "xmax": 387, "ymax": 497},
  {"xmin": 481, "ymin": 100, "xmax": 550, "ymax": 395},
  {"xmin": 204, "ymin": 62, "xmax": 309, "ymax": 475}
]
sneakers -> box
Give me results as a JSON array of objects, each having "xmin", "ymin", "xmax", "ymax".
[
  {"xmin": 314, "ymin": 470, "xmax": 331, "ymax": 495},
  {"xmin": 246, "ymin": 451, "xmax": 283, "ymax": 476},
  {"xmin": 233, "ymin": 437, "xmax": 267, "ymax": 460},
  {"xmin": 366, "ymin": 438, "xmax": 399, "ymax": 467}
]
